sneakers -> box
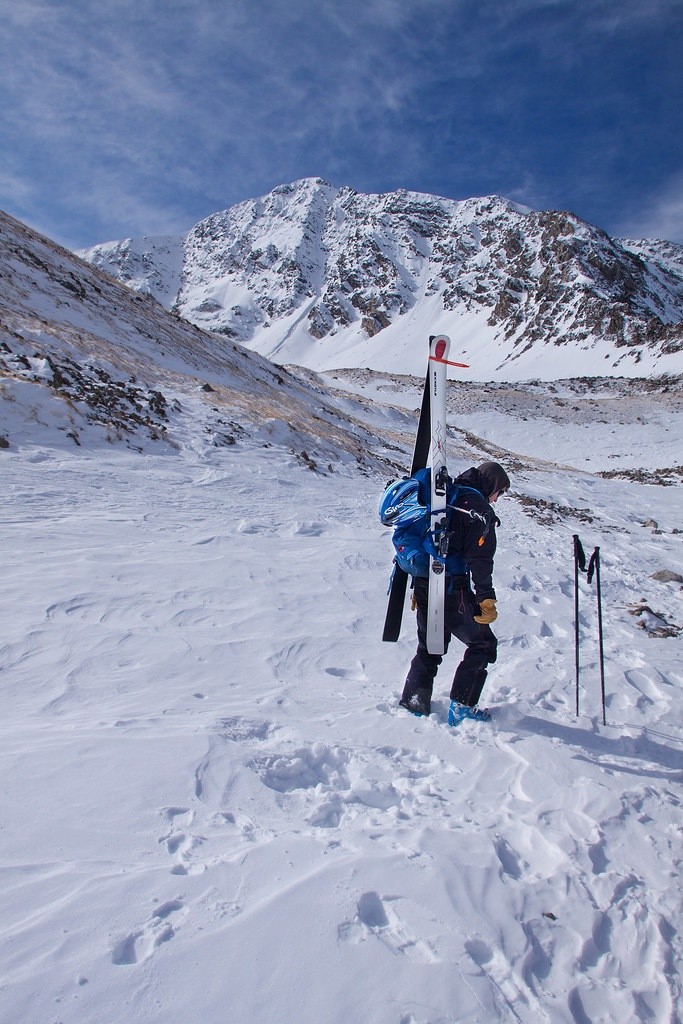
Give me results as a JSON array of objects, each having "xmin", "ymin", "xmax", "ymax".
[
  {"xmin": 448, "ymin": 700, "xmax": 492, "ymax": 727},
  {"xmin": 401, "ymin": 704, "xmax": 428, "ymax": 718}
]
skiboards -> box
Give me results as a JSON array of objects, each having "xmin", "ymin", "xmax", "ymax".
[{"xmin": 382, "ymin": 335, "xmax": 454, "ymax": 655}]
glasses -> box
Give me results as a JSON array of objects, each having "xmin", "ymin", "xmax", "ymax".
[{"xmin": 498, "ymin": 489, "xmax": 504, "ymax": 497}]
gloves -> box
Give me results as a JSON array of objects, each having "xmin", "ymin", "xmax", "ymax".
[
  {"xmin": 410, "ymin": 586, "xmax": 418, "ymax": 611},
  {"xmin": 473, "ymin": 598, "xmax": 498, "ymax": 625}
]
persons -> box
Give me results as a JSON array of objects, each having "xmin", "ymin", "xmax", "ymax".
[{"xmin": 399, "ymin": 462, "xmax": 511, "ymax": 725}]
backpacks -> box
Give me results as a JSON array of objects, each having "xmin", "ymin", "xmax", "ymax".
[{"xmin": 388, "ymin": 468, "xmax": 484, "ymax": 594}]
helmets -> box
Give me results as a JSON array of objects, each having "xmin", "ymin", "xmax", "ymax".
[{"xmin": 378, "ymin": 475, "xmax": 428, "ymax": 528}]
